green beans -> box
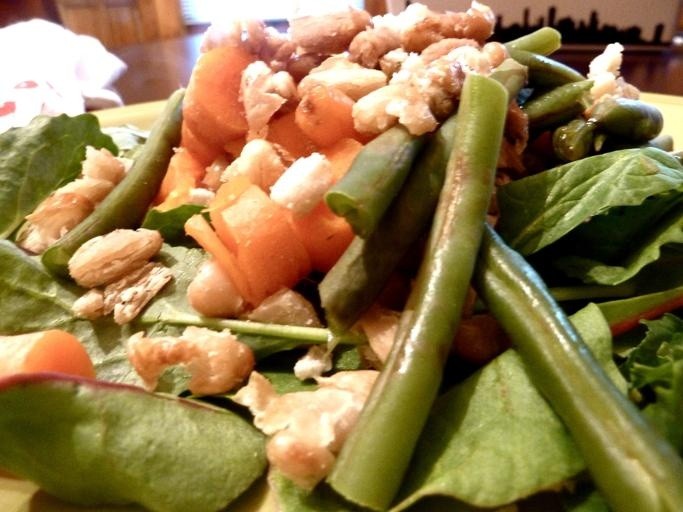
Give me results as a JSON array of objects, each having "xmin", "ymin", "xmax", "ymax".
[
  {"xmin": 318, "ymin": 27, "xmax": 683, "ymax": 512},
  {"xmin": 40, "ymin": 85, "xmax": 186, "ymax": 277}
]
